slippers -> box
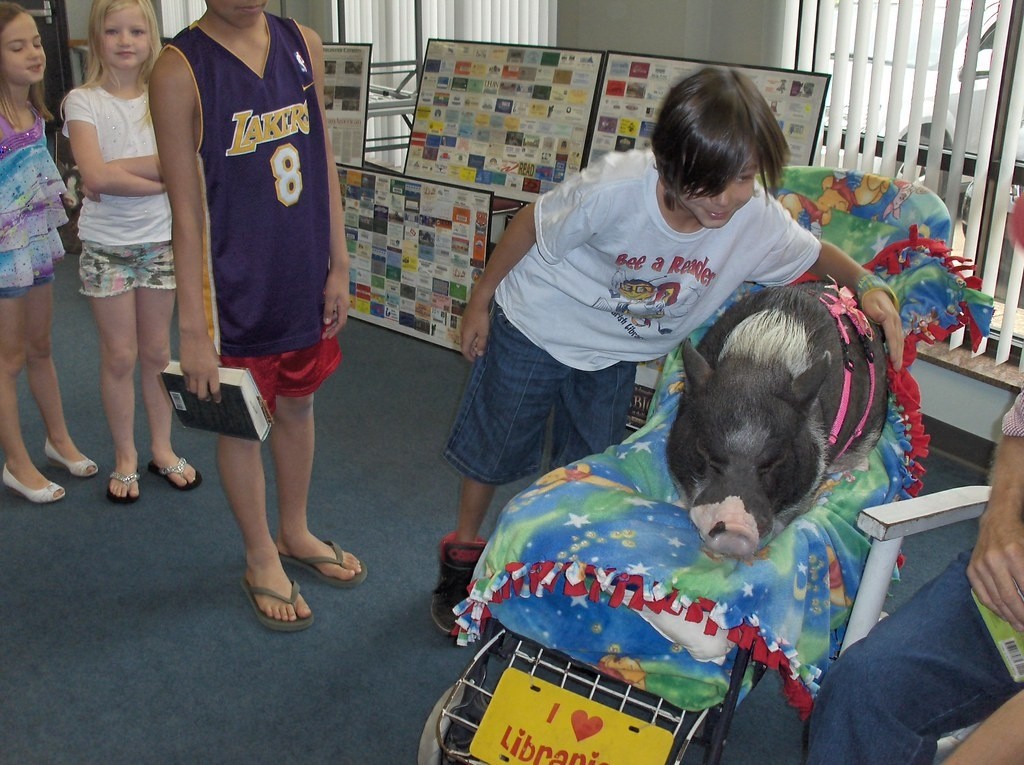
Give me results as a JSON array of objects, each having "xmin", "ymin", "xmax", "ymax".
[
  {"xmin": 106, "ymin": 472, "xmax": 141, "ymax": 507},
  {"xmin": 279, "ymin": 541, "xmax": 368, "ymax": 588},
  {"xmin": 146, "ymin": 457, "xmax": 202, "ymax": 492},
  {"xmin": 240, "ymin": 566, "xmax": 314, "ymax": 632}
]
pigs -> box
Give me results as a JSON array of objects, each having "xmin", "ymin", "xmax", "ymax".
[{"xmin": 664, "ymin": 276, "xmax": 889, "ymax": 556}]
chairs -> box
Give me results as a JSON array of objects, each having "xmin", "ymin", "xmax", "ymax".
[{"xmin": 839, "ymin": 485, "xmax": 988, "ymax": 765}]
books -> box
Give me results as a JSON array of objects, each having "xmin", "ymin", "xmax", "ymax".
[
  {"xmin": 160, "ymin": 361, "xmax": 271, "ymax": 443},
  {"xmin": 970, "ymin": 579, "xmax": 1024, "ymax": 682}
]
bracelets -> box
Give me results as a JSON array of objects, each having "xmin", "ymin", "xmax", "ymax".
[{"xmin": 857, "ymin": 273, "xmax": 900, "ymax": 325}]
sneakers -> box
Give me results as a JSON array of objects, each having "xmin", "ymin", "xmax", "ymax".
[{"xmin": 430, "ymin": 533, "xmax": 489, "ymax": 634}]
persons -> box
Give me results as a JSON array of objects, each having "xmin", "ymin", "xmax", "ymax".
[
  {"xmin": 0, "ymin": 0, "xmax": 99, "ymax": 503},
  {"xmin": 430, "ymin": 68, "xmax": 904, "ymax": 632},
  {"xmin": 805, "ymin": 392, "xmax": 1024, "ymax": 765},
  {"xmin": 148, "ymin": 0, "xmax": 367, "ymax": 631},
  {"xmin": 61, "ymin": 0, "xmax": 202, "ymax": 504}
]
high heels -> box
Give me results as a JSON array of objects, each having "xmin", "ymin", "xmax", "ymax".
[
  {"xmin": 45, "ymin": 436, "xmax": 100, "ymax": 477},
  {"xmin": 2, "ymin": 464, "xmax": 66, "ymax": 504}
]
